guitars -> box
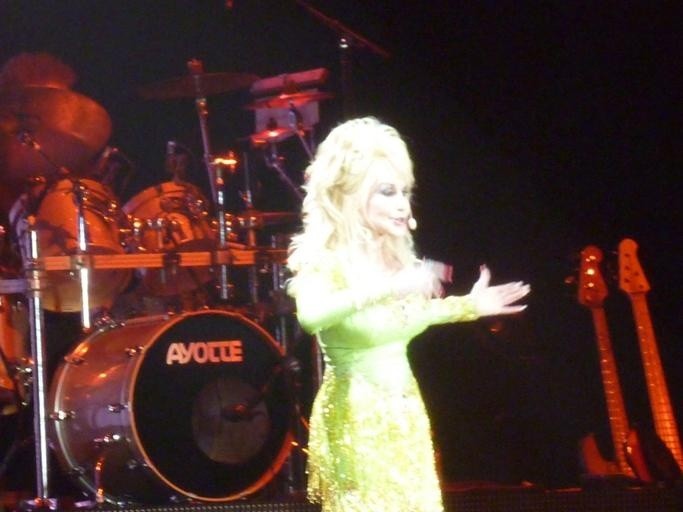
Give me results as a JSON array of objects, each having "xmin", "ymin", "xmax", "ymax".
[{"xmin": 573, "ymin": 238, "xmax": 682, "ymax": 487}]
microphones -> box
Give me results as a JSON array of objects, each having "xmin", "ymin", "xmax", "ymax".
[
  {"xmin": 408, "ymin": 212, "xmax": 418, "ymax": 231},
  {"xmin": 164, "ymin": 141, "xmax": 177, "ymax": 172}
]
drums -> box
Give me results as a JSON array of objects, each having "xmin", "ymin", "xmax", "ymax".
[
  {"xmin": 50, "ymin": 308, "xmax": 300, "ymax": 512},
  {"xmin": 1, "ymin": 263, "xmax": 49, "ymax": 418},
  {"xmin": 7, "ymin": 179, "xmax": 136, "ymax": 336},
  {"xmin": 123, "ymin": 181, "xmax": 221, "ymax": 311}
]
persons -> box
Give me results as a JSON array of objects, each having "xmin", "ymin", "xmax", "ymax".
[
  {"xmin": 119, "ymin": 123, "xmax": 200, "ymax": 207},
  {"xmin": 283, "ymin": 117, "xmax": 534, "ymax": 512}
]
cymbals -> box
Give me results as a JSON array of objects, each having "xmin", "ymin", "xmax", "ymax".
[
  {"xmin": 133, "ymin": 72, "xmax": 261, "ymax": 100},
  {"xmin": 245, "ymin": 92, "xmax": 332, "ymax": 109},
  {"xmin": 229, "ymin": 126, "xmax": 312, "ymax": 145},
  {"xmin": 2, "ymin": 88, "xmax": 113, "ymax": 181}
]
